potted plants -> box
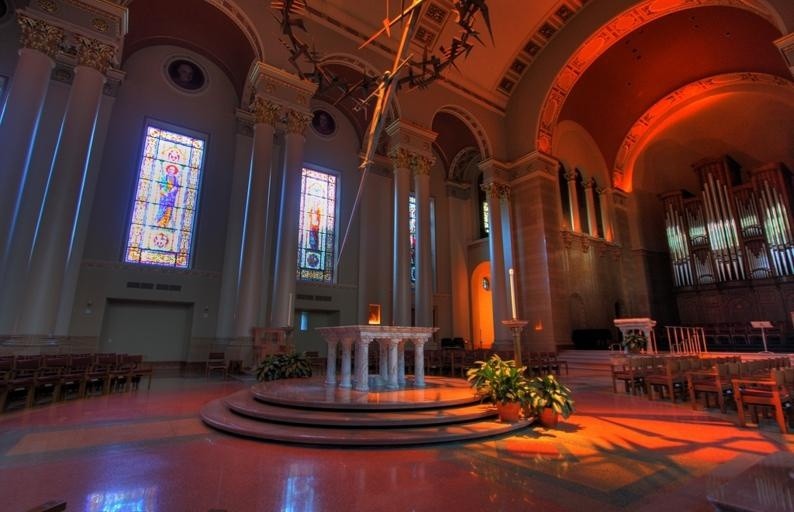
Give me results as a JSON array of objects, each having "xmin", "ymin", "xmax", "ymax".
[
  {"xmin": 464, "ymin": 355, "xmax": 577, "ymax": 428},
  {"xmin": 621, "ymin": 335, "xmax": 648, "ymax": 355}
]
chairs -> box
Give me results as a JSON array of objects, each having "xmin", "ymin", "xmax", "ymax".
[
  {"xmin": 405, "ymin": 347, "xmax": 570, "ymax": 377},
  {"xmin": 609, "ymin": 352, "xmax": 793, "ymax": 435},
  {"xmin": 1, "ymin": 352, "xmax": 153, "ymax": 410}
]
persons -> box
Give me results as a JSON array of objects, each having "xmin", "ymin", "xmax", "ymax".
[{"xmin": 155, "ymin": 165, "xmax": 180, "ymax": 227}]
